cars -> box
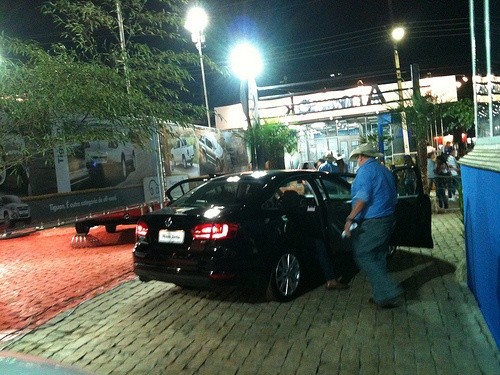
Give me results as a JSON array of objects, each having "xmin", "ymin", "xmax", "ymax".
[{"xmin": 131, "ymin": 163, "xmax": 436, "ymax": 304}]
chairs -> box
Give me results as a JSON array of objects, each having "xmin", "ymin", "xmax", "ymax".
[
  {"xmin": 245, "ymin": 183, "xmax": 263, "ymax": 200},
  {"xmin": 281, "ymin": 190, "xmax": 300, "ymax": 209},
  {"xmin": 213, "ymin": 192, "xmax": 238, "ymax": 203}
]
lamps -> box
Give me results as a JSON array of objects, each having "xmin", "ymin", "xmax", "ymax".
[{"xmin": 455, "ymin": 74, "xmax": 469, "ymax": 83}]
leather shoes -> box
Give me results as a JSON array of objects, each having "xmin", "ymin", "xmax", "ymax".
[{"xmin": 326, "ymin": 278, "xmax": 350, "ymax": 290}]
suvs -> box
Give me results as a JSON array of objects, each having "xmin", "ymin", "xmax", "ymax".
[
  {"xmin": 83, "ymin": 127, "xmax": 137, "ymax": 178},
  {"xmin": 0, "ymin": 195, "xmax": 33, "ymax": 227}
]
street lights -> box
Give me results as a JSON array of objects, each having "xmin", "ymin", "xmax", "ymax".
[
  {"xmin": 183, "ymin": 6, "xmax": 213, "ymax": 128},
  {"xmin": 228, "ymin": 38, "xmax": 264, "ymax": 171},
  {"xmin": 391, "ymin": 25, "xmax": 411, "ymax": 157}
]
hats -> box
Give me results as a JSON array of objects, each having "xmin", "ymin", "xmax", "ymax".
[{"xmin": 349, "ymin": 144, "xmax": 378, "ymax": 160}]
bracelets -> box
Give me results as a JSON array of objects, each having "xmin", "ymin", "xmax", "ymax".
[{"xmin": 346, "ymin": 216, "xmax": 354, "ymax": 222}]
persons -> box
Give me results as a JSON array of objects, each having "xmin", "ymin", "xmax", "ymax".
[
  {"xmin": 283, "ymin": 190, "xmax": 351, "ymax": 289},
  {"xmin": 345, "ymin": 144, "xmax": 405, "ymax": 309},
  {"xmin": 248, "ymin": 142, "xmax": 462, "ymax": 214}
]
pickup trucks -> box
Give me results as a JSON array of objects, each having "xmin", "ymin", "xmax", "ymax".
[
  {"xmin": 198, "ymin": 134, "xmax": 224, "ymax": 166},
  {"xmin": 170, "ymin": 138, "xmax": 194, "ymax": 168}
]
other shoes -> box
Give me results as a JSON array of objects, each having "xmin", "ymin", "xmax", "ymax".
[{"xmin": 369, "ymin": 289, "xmax": 407, "ymax": 308}]
